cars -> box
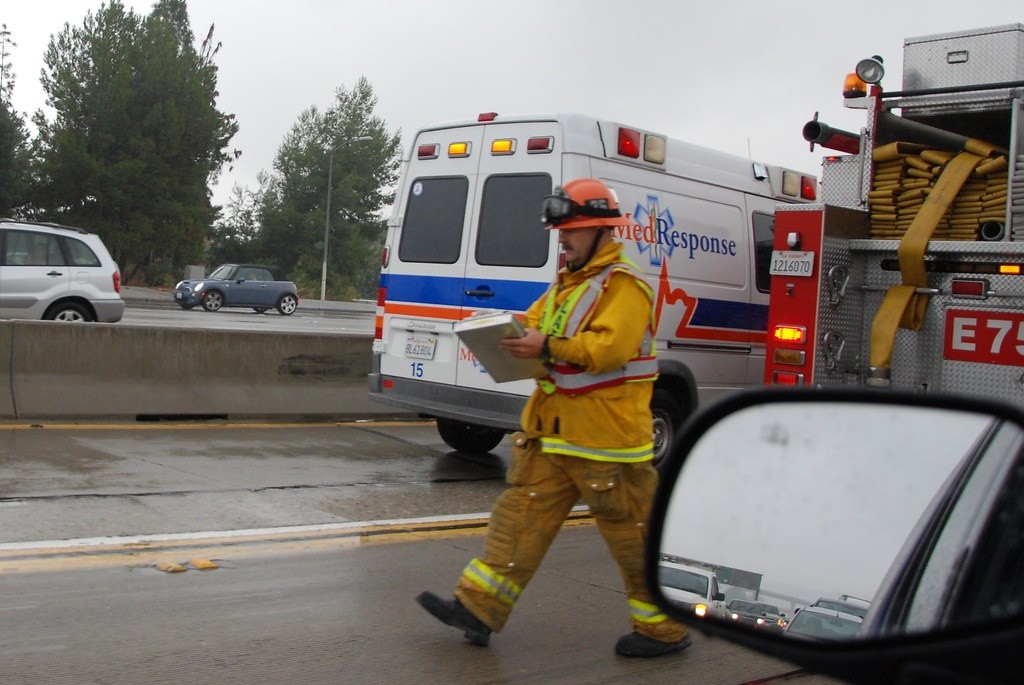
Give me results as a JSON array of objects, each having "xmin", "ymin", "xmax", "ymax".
[
  {"xmin": 657, "ymin": 560, "xmax": 727, "ymax": 619},
  {"xmin": 782, "ymin": 594, "xmax": 872, "ymax": 643},
  {"xmin": 724, "ymin": 599, "xmax": 786, "ymax": 632},
  {"xmin": 174, "ymin": 263, "xmax": 299, "ymax": 315}
]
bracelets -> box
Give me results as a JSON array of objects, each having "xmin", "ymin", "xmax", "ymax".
[{"xmin": 542, "ymin": 333, "xmax": 555, "ymax": 358}]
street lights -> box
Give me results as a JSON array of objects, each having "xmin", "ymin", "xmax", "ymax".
[{"xmin": 320, "ymin": 135, "xmax": 374, "ymax": 302}]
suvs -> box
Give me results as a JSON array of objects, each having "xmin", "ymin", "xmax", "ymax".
[{"xmin": 0, "ymin": 216, "xmax": 126, "ymax": 323}]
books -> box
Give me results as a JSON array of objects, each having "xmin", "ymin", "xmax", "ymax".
[{"xmin": 451, "ymin": 313, "xmax": 550, "ymax": 383}]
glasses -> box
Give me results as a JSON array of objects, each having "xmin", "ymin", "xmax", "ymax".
[{"xmin": 540, "ymin": 197, "xmax": 581, "ymax": 227}]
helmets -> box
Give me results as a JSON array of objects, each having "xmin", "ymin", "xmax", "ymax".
[{"xmin": 545, "ymin": 179, "xmax": 638, "ymax": 230}]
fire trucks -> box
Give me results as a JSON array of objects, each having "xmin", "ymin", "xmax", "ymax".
[{"xmin": 763, "ymin": 22, "xmax": 1024, "ymax": 417}]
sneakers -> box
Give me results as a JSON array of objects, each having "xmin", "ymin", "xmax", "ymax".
[
  {"xmin": 415, "ymin": 591, "xmax": 491, "ymax": 645},
  {"xmin": 611, "ymin": 629, "xmax": 693, "ymax": 655}
]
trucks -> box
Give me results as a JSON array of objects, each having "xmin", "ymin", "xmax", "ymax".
[{"xmin": 368, "ymin": 108, "xmax": 818, "ymax": 456}]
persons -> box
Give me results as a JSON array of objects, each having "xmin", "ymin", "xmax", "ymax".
[
  {"xmin": 689, "ymin": 580, "xmax": 701, "ymax": 591},
  {"xmin": 25, "ymin": 243, "xmax": 49, "ymax": 265},
  {"xmin": 802, "ymin": 618, "xmax": 822, "ymax": 635},
  {"xmin": 416, "ymin": 178, "xmax": 693, "ymax": 658}
]
